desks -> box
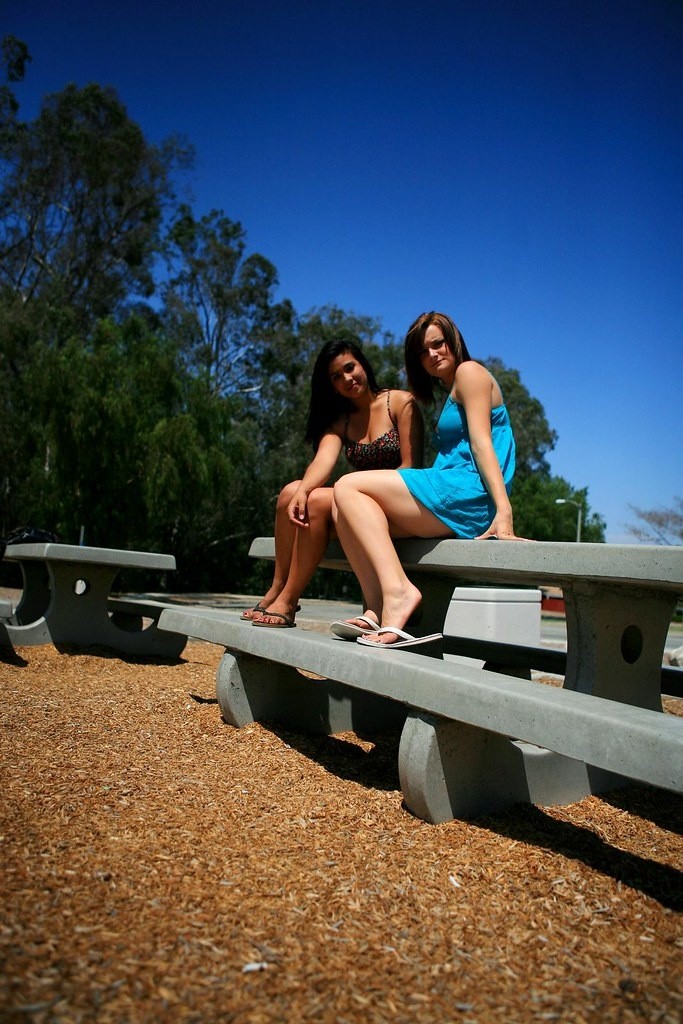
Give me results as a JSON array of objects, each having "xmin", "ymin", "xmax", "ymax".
[
  {"xmin": 216, "ymin": 535, "xmax": 683, "ymax": 822},
  {"xmin": 3, "ymin": 543, "xmax": 188, "ymax": 659}
]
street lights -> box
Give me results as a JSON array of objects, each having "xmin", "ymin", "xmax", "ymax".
[{"xmin": 554, "ymin": 498, "xmax": 582, "ymax": 543}]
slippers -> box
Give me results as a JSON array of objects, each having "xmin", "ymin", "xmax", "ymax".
[
  {"xmin": 240, "ymin": 603, "xmax": 301, "ymax": 620},
  {"xmin": 330, "ymin": 616, "xmax": 380, "ymax": 639},
  {"xmin": 252, "ymin": 609, "xmax": 297, "ymax": 628},
  {"xmin": 357, "ymin": 626, "xmax": 443, "ymax": 647}
]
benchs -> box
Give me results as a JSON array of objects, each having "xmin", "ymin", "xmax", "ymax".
[
  {"xmin": 441, "ymin": 633, "xmax": 683, "ymax": 698},
  {"xmin": 156, "ymin": 609, "xmax": 683, "ymax": 795},
  {"xmin": 107, "ymin": 598, "xmax": 192, "ymax": 619},
  {"xmin": 0, "ymin": 600, "xmax": 12, "ymax": 618}
]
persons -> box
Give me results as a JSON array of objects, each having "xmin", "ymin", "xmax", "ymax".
[
  {"xmin": 332, "ymin": 312, "xmax": 537, "ymax": 648},
  {"xmin": 238, "ymin": 336, "xmax": 425, "ymax": 627}
]
